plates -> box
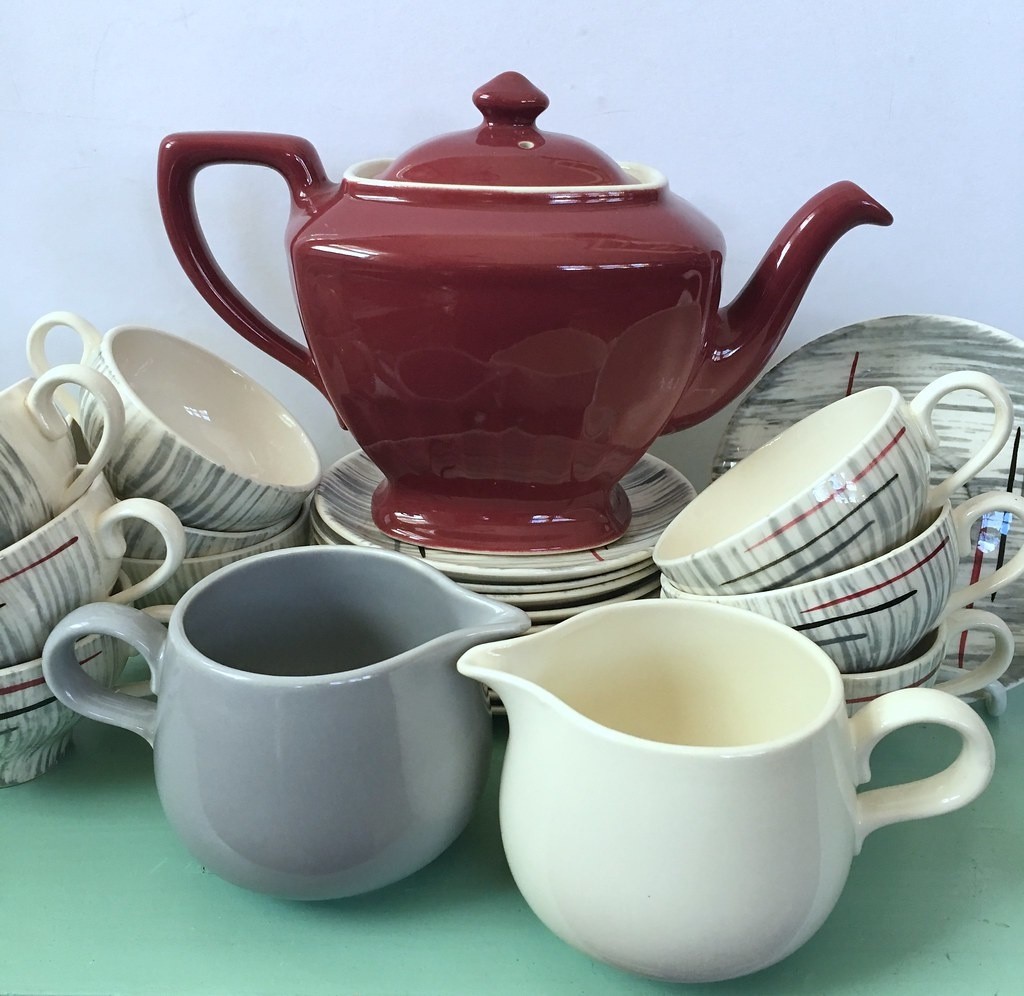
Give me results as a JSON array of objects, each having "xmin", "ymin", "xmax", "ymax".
[
  {"xmin": 311, "ymin": 438, "xmax": 693, "ymax": 718},
  {"xmin": 714, "ymin": 312, "xmax": 1024, "ymax": 696}
]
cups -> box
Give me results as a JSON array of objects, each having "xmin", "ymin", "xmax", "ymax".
[
  {"xmin": 0, "ymin": 308, "xmax": 311, "ymax": 791},
  {"xmin": 654, "ymin": 369, "xmax": 1024, "ymax": 718}
]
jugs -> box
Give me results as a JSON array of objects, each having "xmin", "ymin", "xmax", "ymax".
[
  {"xmin": 35, "ymin": 543, "xmax": 524, "ymax": 902},
  {"xmin": 461, "ymin": 600, "xmax": 993, "ymax": 984}
]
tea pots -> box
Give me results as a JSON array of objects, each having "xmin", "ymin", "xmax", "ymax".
[{"xmin": 154, "ymin": 73, "xmax": 893, "ymax": 557}]
